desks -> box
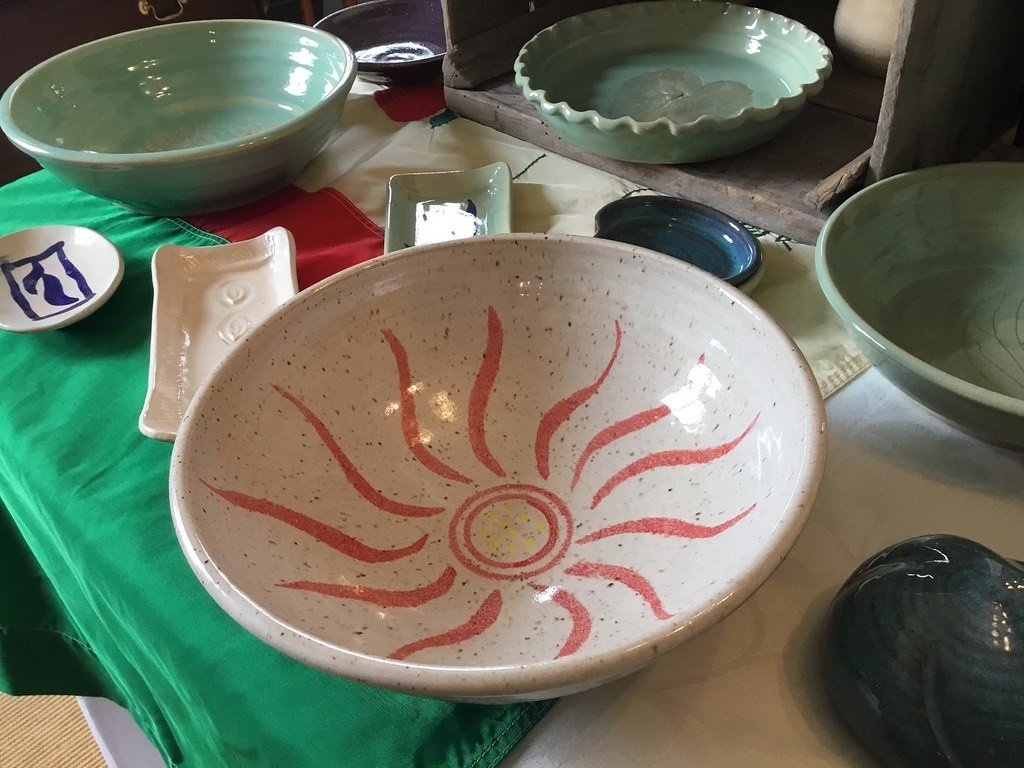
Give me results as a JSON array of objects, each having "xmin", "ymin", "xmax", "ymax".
[{"xmin": 2, "ymin": 73, "xmax": 1024, "ymax": 767}]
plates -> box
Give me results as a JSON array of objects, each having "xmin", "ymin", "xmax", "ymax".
[
  {"xmin": 381, "ymin": 162, "xmax": 515, "ymax": 253},
  {"xmin": 593, "ymin": 197, "xmax": 766, "ymax": 294},
  {"xmin": 0, "ymin": 226, "xmax": 125, "ymax": 333},
  {"xmin": 139, "ymin": 226, "xmax": 304, "ymax": 445}
]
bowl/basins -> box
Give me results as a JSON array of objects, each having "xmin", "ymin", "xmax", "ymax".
[
  {"xmin": 513, "ymin": 0, "xmax": 838, "ymax": 163},
  {"xmin": 166, "ymin": 230, "xmax": 830, "ymax": 707},
  {"xmin": 813, "ymin": 160, "xmax": 1022, "ymax": 451},
  {"xmin": 0, "ymin": 20, "xmax": 355, "ymax": 223},
  {"xmin": 827, "ymin": 533, "xmax": 1024, "ymax": 767},
  {"xmin": 312, "ymin": 1, "xmax": 447, "ymax": 86}
]
